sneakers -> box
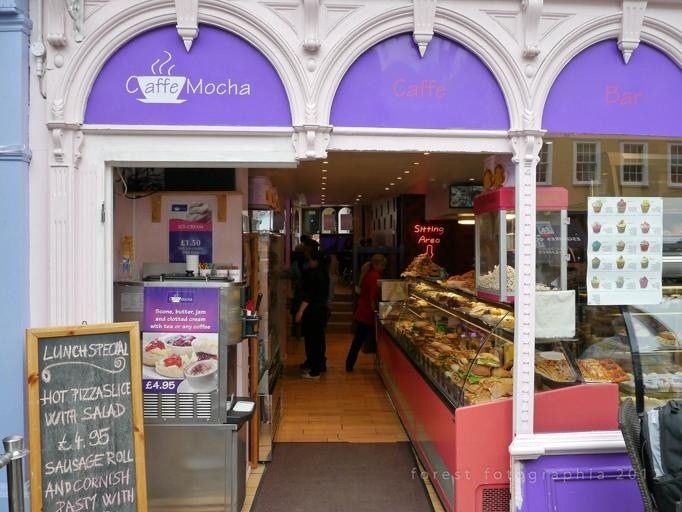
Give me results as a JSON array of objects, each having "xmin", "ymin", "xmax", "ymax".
[{"xmin": 296, "ymin": 363, "xmax": 327, "ymax": 380}]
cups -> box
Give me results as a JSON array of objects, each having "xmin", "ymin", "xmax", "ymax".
[
  {"xmin": 183, "ymin": 359, "xmax": 219, "ymax": 390},
  {"xmin": 217, "ymin": 269, "xmax": 228, "ymax": 277},
  {"xmin": 229, "ymin": 269, "xmax": 240, "ymax": 282}
]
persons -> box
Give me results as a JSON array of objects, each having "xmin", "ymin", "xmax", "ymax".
[
  {"xmin": 345, "ymin": 253, "xmax": 386, "ymax": 372},
  {"xmin": 290, "ymin": 234, "xmax": 333, "ymax": 379},
  {"xmin": 351, "ymin": 257, "xmax": 388, "ymax": 319}
]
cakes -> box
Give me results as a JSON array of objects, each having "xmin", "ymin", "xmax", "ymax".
[
  {"xmin": 591, "ymin": 199, "xmax": 650, "ymax": 289},
  {"xmin": 142, "ymin": 334, "xmax": 217, "ymax": 377}
]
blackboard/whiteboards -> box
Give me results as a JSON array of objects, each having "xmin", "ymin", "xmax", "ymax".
[{"xmin": 25, "ymin": 320, "xmax": 149, "ymax": 512}]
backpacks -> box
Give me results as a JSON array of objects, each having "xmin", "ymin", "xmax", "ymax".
[{"xmin": 641, "ymin": 399, "xmax": 682, "ymax": 512}]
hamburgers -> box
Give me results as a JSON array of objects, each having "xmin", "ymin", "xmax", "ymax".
[{"xmin": 445, "ymin": 351, "xmax": 511, "ymax": 398}]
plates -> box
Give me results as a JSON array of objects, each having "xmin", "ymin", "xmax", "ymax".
[{"xmin": 177, "ymin": 379, "xmax": 217, "ymax": 394}]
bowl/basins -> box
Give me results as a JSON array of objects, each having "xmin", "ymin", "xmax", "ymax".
[{"xmin": 185, "ymin": 255, "xmax": 199, "ymax": 276}]
